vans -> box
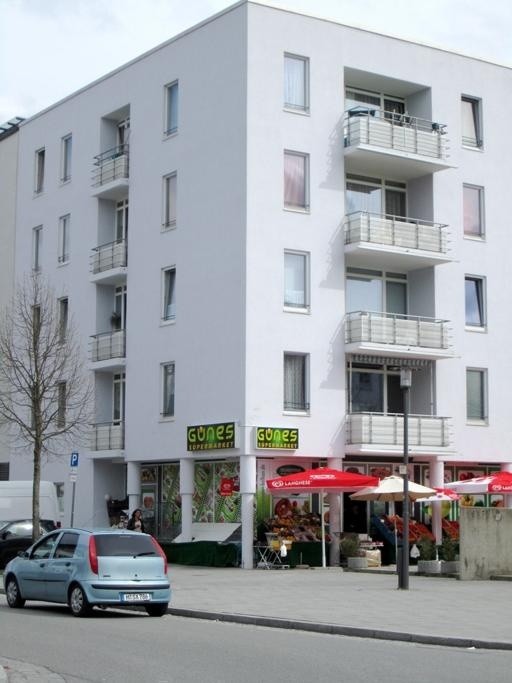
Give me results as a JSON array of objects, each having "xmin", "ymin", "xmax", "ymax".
[{"xmin": 0, "ymin": 479, "xmax": 62, "ymax": 529}]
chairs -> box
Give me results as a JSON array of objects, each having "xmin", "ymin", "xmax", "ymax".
[{"xmin": 252, "ymin": 532, "xmax": 286, "ymax": 569}]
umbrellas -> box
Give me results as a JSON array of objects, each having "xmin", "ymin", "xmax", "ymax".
[
  {"xmin": 411, "ymin": 485, "xmax": 463, "ymax": 561},
  {"xmin": 265, "ymin": 466, "xmax": 382, "ymax": 567},
  {"xmin": 435, "ymin": 468, "xmax": 511, "ymax": 508},
  {"xmin": 349, "ymin": 473, "xmax": 438, "ymax": 566}
]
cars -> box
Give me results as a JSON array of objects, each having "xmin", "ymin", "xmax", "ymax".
[
  {"xmin": 3, "ymin": 526, "xmax": 173, "ymax": 618},
  {"xmin": 1, "ymin": 518, "xmax": 59, "ymax": 569}
]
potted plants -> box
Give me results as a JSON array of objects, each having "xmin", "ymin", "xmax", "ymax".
[
  {"xmin": 416, "ymin": 532, "xmax": 460, "ymax": 574},
  {"xmin": 342, "ymin": 535, "xmax": 369, "ymax": 570}
]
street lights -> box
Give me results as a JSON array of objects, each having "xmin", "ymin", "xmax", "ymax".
[{"xmin": 390, "ymin": 363, "xmax": 424, "ymax": 592}]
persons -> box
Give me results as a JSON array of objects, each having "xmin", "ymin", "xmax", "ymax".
[{"xmin": 125, "ymin": 508, "xmax": 144, "ymax": 533}]
